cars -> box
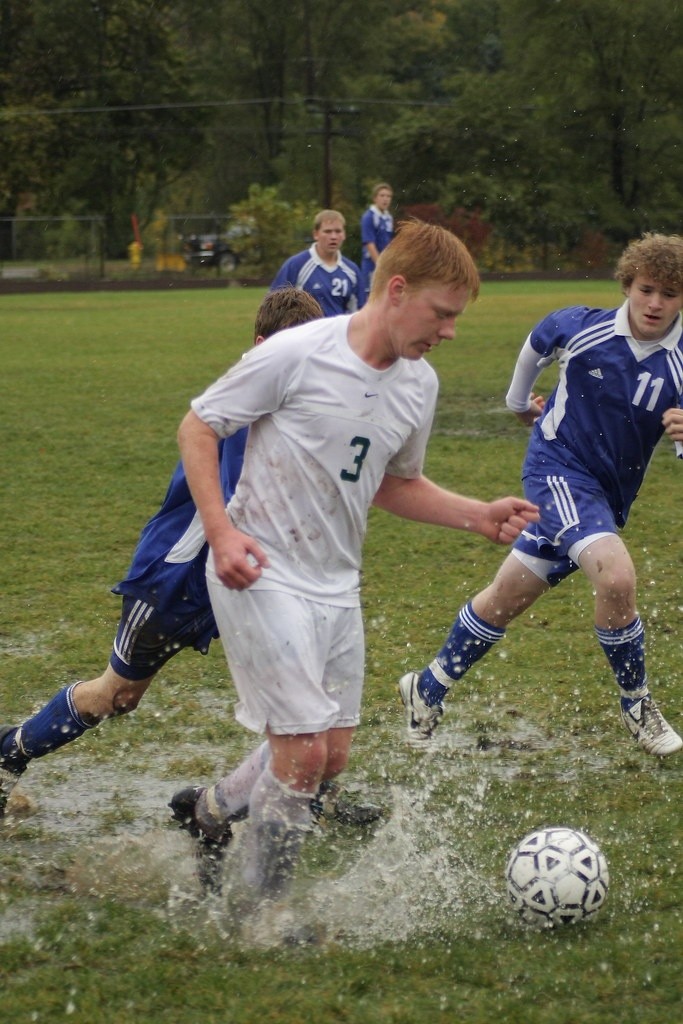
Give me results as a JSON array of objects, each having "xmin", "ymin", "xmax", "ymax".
[{"xmin": 179, "ymin": 218, "xmax": 255, "ymax": 274}]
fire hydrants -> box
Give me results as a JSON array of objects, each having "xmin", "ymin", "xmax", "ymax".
[{"xmin": 125, "ymin": 241, "xmax": 146, "ymax": 270}]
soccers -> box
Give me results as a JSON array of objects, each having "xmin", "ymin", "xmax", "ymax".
[{"xmin": 503, "ymin": 825, "xmax": 612, "ymax": 932}]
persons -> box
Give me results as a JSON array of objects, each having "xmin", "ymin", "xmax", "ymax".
[
  {"xmin": 169, "ymin": 215, "xmax": 539, "ymax": 926},
  {"xmin": 397, "ymin": 236, "xmax": 683, "ymax": 755},
  {"xmin": 360, "ymin": 183, "xmax": 396, "ymax": 277},
  {"xmin": 0, "ymin": 285, "xmax": 386, "ymax": 898},
  {"xmin": 271, "ymin": 208, "xmax": 370, "ymax": 318}
]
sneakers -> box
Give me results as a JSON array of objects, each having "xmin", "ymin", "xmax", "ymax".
[
  {"xmin": 307, "ymin": 780, "xmax": 384, "ymax": 828},
  {"xmin": 620, "ymin": 693, "xmax": 683, "ymax": 756},
  {"xmin": 168, "ymin": 784, "xmax": 235, "ymax": 897},
  {"xmin": 0, "ymin": 724, "xmax": 30, "ymax": 816},
  {"xmin": 396, "ymin": 671, "xmax": 443, "ymax": 753}
]
